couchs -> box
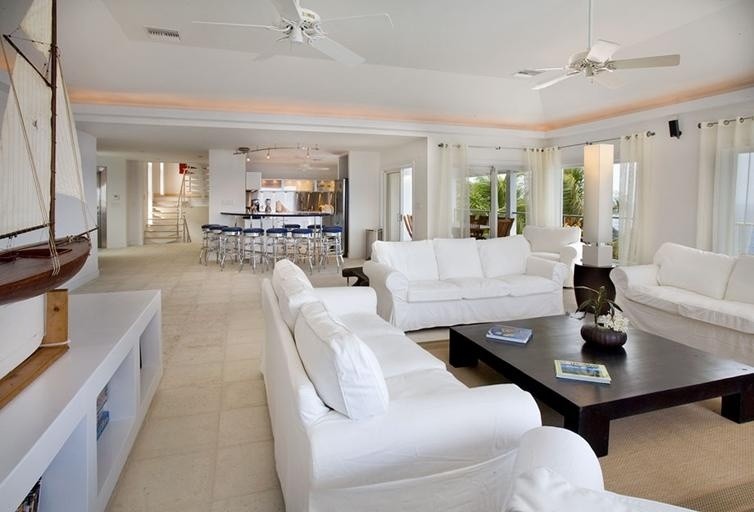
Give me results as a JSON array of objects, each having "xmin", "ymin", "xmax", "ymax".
[
  {"xmin": 609, "ymin": 242, "xmax": 754, "ymax": 367},
  {"xmin": 261, "ymin": 279, "xmax": 546, "ymax": 512},
  {"xmin": 363, "ymin": 236, "xmax": 569, "ymax": 331}
]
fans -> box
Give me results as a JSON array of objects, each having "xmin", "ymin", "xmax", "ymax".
[
  {"xmin": 525, "ymin": 0, "xmax": 680, "ymax": 91},
  {"xmin": 192, "ymin": 0, "xmax": 394, "ymax": 67}
]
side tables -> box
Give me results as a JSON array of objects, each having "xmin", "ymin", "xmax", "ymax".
[{"xmin": 574, "ymin": 264, "xmax": 616, "ymax": 314}]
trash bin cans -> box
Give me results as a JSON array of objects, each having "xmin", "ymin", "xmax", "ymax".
[{"xmin": 366, "ymin": 228, "xmax": 382, "ymax": 260}]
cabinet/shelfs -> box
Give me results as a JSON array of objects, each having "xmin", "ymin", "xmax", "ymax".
[{"xmin": 0, "ymin": 290, "xmax": 163, "ymax": 512}]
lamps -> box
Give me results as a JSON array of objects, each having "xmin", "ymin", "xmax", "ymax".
[{"xmin": 233, "ymin": 144, "xmax": 320, "ymax": 162}]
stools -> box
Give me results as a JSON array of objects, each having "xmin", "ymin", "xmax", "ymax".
[{"xmin": 199, "ymin": 224, "xmax": 345, "ymax": 274}]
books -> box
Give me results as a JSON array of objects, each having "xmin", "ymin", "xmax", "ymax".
[
  {"xmin": 552, "ymin": 357, "xmax": 612, "ymax": 385},
  {"xmin": 485, "ymin": 323, "xmax": 535, "ymax": 347}
]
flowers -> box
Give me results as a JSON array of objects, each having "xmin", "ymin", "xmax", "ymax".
[{"xmin": 598, "ymin": 312, "xmax": 628, "ymax": 334}]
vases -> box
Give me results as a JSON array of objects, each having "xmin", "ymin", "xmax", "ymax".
[
  {"xmin": 580, "ymin": 323, "xmax": 627, "ymax": 346},
  {"xmin": 581, "ymin": 341, "xmax": 626, "ymax": 366}
]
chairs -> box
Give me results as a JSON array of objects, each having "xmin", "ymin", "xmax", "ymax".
[
  {"xmin": 516, "ymin": 226, "xmax": 584, "ymax": 289},
  {"xmin": 503, "ymin": 428, "xmax": 697, "ymax": 512}
]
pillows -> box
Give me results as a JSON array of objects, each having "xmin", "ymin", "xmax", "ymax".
[
  {"xmin": 271, "ymin": 259, "xmax": 318, "ymax": 331},
  {"xmin": 659, "ymin": 251, "xmax": 736, "ymax": 300},
  {"xmin": 725, "ymin": 254, "xmax": 754, "ymax": 304},
  {"xmin": 372, "ymin": 235, "xmax": 528, "ymax": 281},
  {"xmin": 295, "ymin": 300, "xmax": 391, "ymax": 418}
]
